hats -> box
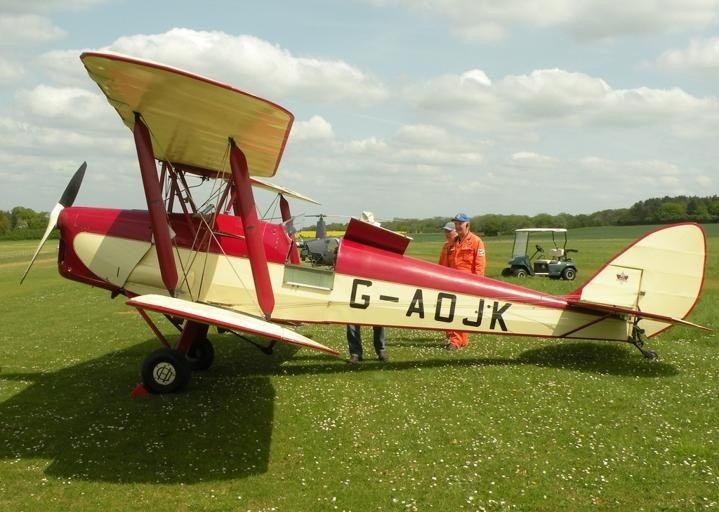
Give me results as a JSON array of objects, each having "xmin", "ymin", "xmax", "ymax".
[
  {"xmin": 443, "ymin": 221, "xmax": 456, "ymax": 231},
  {"xmin": 451, "ymin": 213, "xmax": 470, "ymax": 222},
  {"xmin": 360, "ymin": 210, "xmax": 381, "ymax": 227}
]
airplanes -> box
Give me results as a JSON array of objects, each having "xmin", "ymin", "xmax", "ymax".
[{"xmin": 20, "ymin": 51, "xmax": 714, "ymax": 392}]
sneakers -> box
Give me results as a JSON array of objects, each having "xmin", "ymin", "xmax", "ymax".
[
  {"xmin": 446, "ymin": 343, "xmax": 458, "ymax": 351},
  {"xmin": 348, "ymin": 351, "xmax": 388, "ymax": 366}
]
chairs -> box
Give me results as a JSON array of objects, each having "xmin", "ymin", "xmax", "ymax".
[{"xmin": 533, "ymin": 248, "xmax": 566, "ymax": 268}]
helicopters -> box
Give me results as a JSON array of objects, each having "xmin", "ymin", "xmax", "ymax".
[{"xmin": 262, "ymin": 212, "xmax": 350, "ymax": 269}]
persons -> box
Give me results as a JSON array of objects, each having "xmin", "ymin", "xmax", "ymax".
[
  {"xmin": 346, "ymin": 325, "xmax": 388, "ymax": 363},
  {"xmin": 438, "ymin": 214, "xmax": 486, "ymax": 351}
]
